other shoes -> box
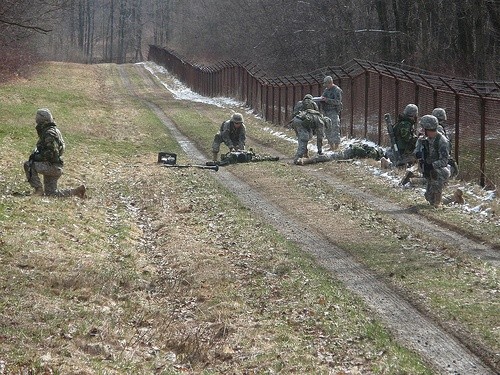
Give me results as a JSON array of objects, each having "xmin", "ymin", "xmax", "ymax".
[
  {"xmin": 334, "ymin": 144, "xmax": 339, "ymax": 152},
  {"xmin": 398, "ymin": 171, "xmax": 414, "ymax": 185},
  {"xmin": 326, "ymin": 144, "xmax": 334, "ymax": 151},
  {"xmin": 29, "ymin": 188, "xmax": 44, "ymax": 197},
  {"xmin": 379, "ymin": 157, "xmax": 392, "ymax": 169},
  {"xmin": 455, "ymin": 187, "xmax": 465, "ymax": 204},
  {"xmin": 72, "ymin": 185, "xmax": 86, "ymax": 198}
]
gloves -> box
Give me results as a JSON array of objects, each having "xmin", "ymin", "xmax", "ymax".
[
  {"xmin": 425, "ymin": 162, "xmax": 432, "ymax": 169},
  {"xmin": 29, "ymin": 153, "xmax": 36, "ymax": 163},
  {"xmin": 416, "ymin": 151, "xmax": 421, "ymax": 160}
]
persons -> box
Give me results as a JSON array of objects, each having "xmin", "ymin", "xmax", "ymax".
[
  {"xmin": 24, "ymin": 108, "xmax": 86, "ymax": 199},
  {"xmin": 290, "ymin": 75, "xmax": 343, "ymax": 164},
  {"xmin": 390, "ymin": 104, "xmax": 417, "ymax": 168},
  {"xmin": 401, "ymin": 108, "xmax": 450, "ymax": 186},
  {"xmin": 212, "ymin": 113, "xmax": 246, "ymax": 160},
  {"xmin": 413, "ymin": 115, "xmax": 464, "ymax": 208}
]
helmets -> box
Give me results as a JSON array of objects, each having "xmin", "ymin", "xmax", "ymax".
[
  {"xmin": 35, "ymin": 109, "xmax": 53, "ymax": 122},
  {"xmin": 303, "ymin": 94, "xmax": 313, "ymax": 100},
  {"xmin": 404, "ymin": 104, "xmax": 419, "ymax": 116},
  {"xmin": 432, "ymin": 109, "xmax": 447, "ymax": 121},
  {"xmin": 323, "ymin": 117, "xmax": 333, "ymax": 129},
  {"xmin": 419, "ymin": 114, "xmax": 439, "ymax": 129},
  {"xmin": 231, "ymin": 113, "xmax": 244, "ymax": 124}
]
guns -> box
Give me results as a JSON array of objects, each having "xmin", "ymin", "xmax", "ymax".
[
  {"xmin": 161, "ymin": 163, "xmax": 219, "ymax": 172},
  {"xmin": 384, "ymin": 112, "xmax": 398, "ymax": 152}
]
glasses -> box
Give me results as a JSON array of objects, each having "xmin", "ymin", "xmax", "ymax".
[{"xmin": 427, "ymin": 127, "xmax": 436, "ymax": 132}]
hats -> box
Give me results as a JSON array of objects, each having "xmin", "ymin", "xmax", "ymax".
[{"xmin": 323, "ymin": 76, "xmax": 333, "ymax": 87}]
